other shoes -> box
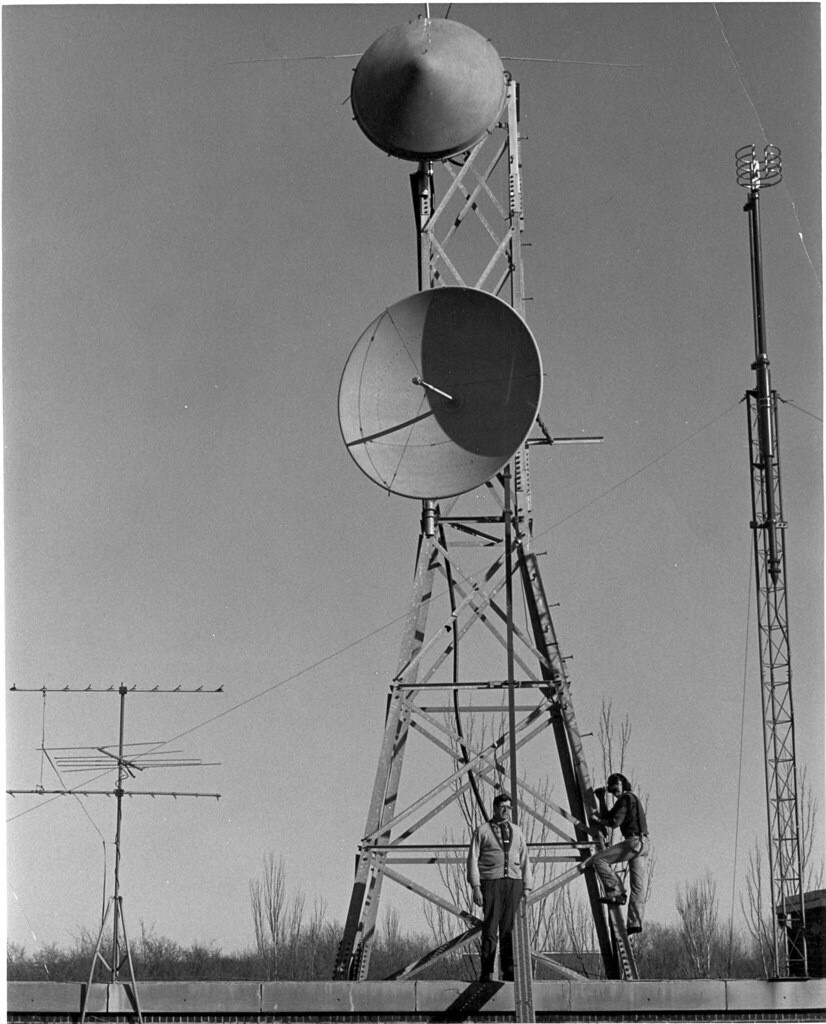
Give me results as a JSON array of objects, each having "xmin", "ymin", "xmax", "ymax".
[
  {"xmin": 627, "ymin": 927, "xmax": 642, "ymax": 936},
  {"xmin": 502, "ymin": 969, "xmax": 514, "ymax": 982},
  {"xmin": 480, "ymin": 973, "xmax": 491, "ymax": 983},
  {"xmin": 597, "ymin": 895, "xmax": 628, "ymax": 905}
]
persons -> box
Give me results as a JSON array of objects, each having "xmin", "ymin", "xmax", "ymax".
[
  {"xmin": 465, "ymin": 793, "xmax": 534, "ymax": 982},
  {"xmin": 592, "ymin": 773, "xmax": 650, "ymax": 936}
]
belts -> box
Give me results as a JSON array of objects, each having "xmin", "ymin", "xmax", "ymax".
[{"xmin": 629, "ymin": 833, "xmax": 648, "ymax": 837}]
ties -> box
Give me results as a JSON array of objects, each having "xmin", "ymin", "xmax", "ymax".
[{"xmin": 499, "ymin": 823, "xmax": 509, "ymax": 851}]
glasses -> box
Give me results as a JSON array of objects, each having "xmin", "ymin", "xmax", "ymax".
[{"xmin": 499, "ymin": 804, "xmax": 513, "ymax": 810}]
